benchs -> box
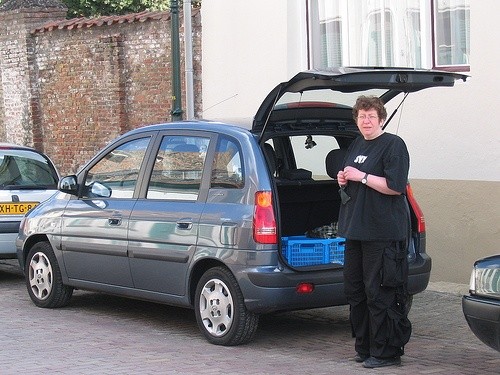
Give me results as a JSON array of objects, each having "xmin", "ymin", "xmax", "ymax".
[{"xmin": 263, "ymin": 143, "xmax": 348, "ymax": 237}]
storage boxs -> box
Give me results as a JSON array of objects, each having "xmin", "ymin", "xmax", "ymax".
[{"xmin": 280, "ymin": 235, "xmax": 346, "ymax": 268}]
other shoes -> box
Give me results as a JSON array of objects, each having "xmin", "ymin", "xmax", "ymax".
[{"xmin": 356, "ymin": 355, "xmax": 401, "ymax": 367}]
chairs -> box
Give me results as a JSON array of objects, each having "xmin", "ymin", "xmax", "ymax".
[{"xmin": 166, "ymin": 143, "xmax": 204, "ymax": 183}]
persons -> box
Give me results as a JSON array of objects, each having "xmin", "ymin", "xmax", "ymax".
[{"xmin": 337, "ymin": 95, "xmax": 412, "ymax": 368}]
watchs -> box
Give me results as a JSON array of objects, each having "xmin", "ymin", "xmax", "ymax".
[{"xmin": 362, "ymin": 173, "xmax": 368, "ymax": 184}]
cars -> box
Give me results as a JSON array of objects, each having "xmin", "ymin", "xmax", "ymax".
[
  {"xmin": 15, "ymin": 63, "xmax": 472, "ymax": 347},
  {"xmin": 461, "ymin": 254, "xmax": 500, "ymax": 352},
  {"xmin": 0, "ymin": 142, "xmax": 64, "ymax": 259}
]
